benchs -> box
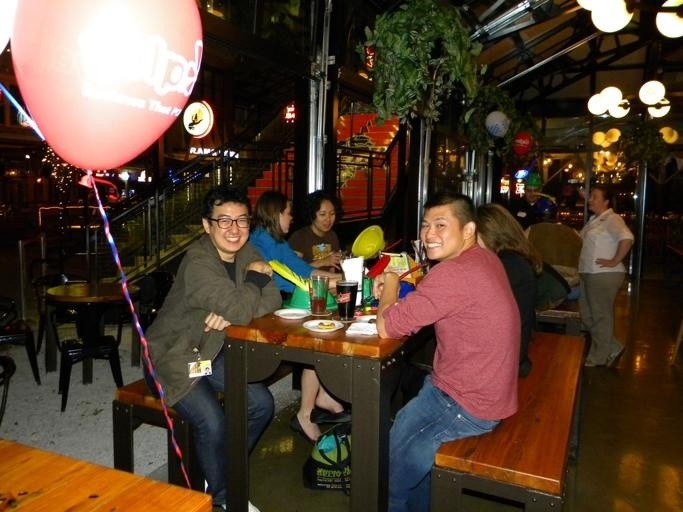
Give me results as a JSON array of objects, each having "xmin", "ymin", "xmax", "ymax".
[
  {"xmin": 426, "ymin": 330, "xmax": 587, "ymax": 511},
  {"xmin": 532, "ymin": 299, "xmax": 582, "ymax": 336},
  {"xmin": 110, "ymin": 360, "xmax": 293, "ymax": 491}
]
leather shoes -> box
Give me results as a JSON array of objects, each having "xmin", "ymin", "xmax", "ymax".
[
  {"xmin": 289, "ymin": 413, "xmax": 324, "ymax": 445},
  {"xmin": 310, "ymin": 408, "xmax": 352, "ymax": 424}
]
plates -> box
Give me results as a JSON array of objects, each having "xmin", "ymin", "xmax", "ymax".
[
  {"xmin": 273, "ymin": 308, "xmax": 312, "ymax": 319},
  {"xmin": 303, "ymin": 319, "xmax": 345, "ymax": 333},
  {"xmin": 357, "ymin": 315, "xmax": 378, "ymax": 324}
]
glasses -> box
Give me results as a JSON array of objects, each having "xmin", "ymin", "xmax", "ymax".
[{"xmin": 208, "ymin": 217, "xmax": 253, "ymax": 229}]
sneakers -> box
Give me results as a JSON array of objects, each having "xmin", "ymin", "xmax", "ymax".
[
  {"xmin": 584, "ymin": 343, "xmax": 625, "ymax": 369},
  {"xmin": 221, "ymin": 500, "xmax": 260, "ymax": 512}
]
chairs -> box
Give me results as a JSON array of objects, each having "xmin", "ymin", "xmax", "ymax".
[
  {"xmin": 29, "ymin": 257, "xmax": 82, "ymax": 355},
  {"xmin": 0, "ymin": 354, "xmax": 17, "ymax": 428},
  {"xmin": 0, "ymin": 295, "xmax": 42, "ymax": 385},
  {"xmin": 50, "ymin": 301, "xmax": 128, "ymax": 412}
]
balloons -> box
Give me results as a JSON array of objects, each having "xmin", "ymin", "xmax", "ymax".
[
  {"xmin": 511, "ymin": 130, "xmax": 533, "ymax": 158},
  {"xmin": 484, "ymin": 110, "xmax": 511, "ymax": 139},
  {"xmin": 8, "ymin": 0, "xmax": 205, "ymax": 191}
]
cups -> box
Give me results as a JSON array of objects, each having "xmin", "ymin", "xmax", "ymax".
[
  {"xmin": 336, "ymin": 279, "xmax": 359, "ymax": 319},
  {"xmin": 334, "ymin": 249, "xmax": 345, "ymax": 261},
  {"xmin": 307, "ymin": 275, "xmax": 328, "ymax": 314}
]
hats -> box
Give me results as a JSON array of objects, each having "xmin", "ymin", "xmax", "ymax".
[{"xmin": 525, "ymin": 173, "xmax": 544, "ymax": 191}]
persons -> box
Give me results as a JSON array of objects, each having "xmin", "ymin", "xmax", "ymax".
[
  {"xmin": 371, "ymin": 189, "xmax": 521, "ymax": 512},
  {"xmin": 523, "ymin": 198, "xmax": 584, "ymax": 301},
  {"xmin": 140, "ymin": 184, "xmax": 276, "ymax": 512},
  {"xmin": 509, "ymin": 174, "xmax": 557, "ymax": 230},
  {"xmin": 247, "ymin": 189, "xmax": 346, "ymax": 446},
  {"xmin": 575, "ymin": 185, "xmax": 635, "ymax": 375},
  {"xmin": 288, "ymin": 190, "xmax": 344, "ymax": 275},
  {"xmin": 474, "ymin": 202, "xmax": 543, "ymax": 378}
]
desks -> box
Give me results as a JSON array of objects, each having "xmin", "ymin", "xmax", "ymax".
[
  {"xmin": 222, "ymin": 298, "xmax": 434, "ymax": 511},
  {"xmin": 0, "ymin": 437, "xmax": 213, "ymax": 512},
  {"xmin": 46, "ymin": 281, "xmax": 140, "ymax": 386}
]
